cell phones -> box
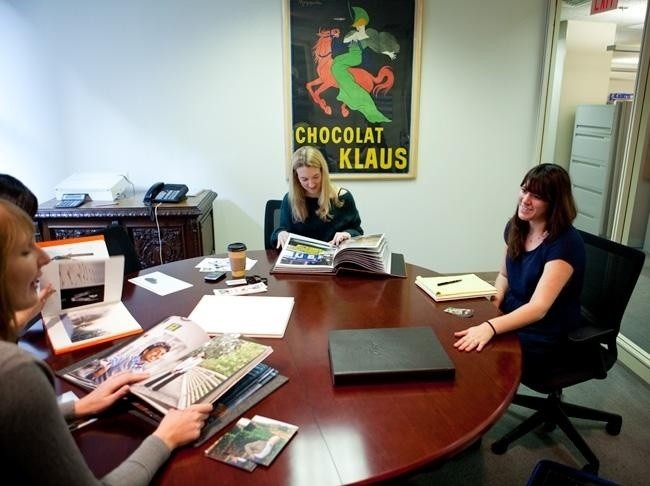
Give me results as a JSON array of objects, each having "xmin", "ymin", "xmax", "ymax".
[{"xmin": 203, "ymin": 271, "xmax": 226, "ymax": 282}]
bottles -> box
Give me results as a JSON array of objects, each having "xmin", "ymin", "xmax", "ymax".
[{"xmin": 55, "ymin": 188, "xmax": 62, "ymax": 200}]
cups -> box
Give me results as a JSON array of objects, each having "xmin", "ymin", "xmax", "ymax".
[{"xmin": 227, "ymin": 241, "xmax": 248, "ymax": 278}]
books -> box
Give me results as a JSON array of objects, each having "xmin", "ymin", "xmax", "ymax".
[
  {"xmin": 327, "ymin": 326, "xmax": 455, "ymax": 387},
  {"xmin": 414, "ymin": 273, "xmax": 498, "ymax": 303},
  {"xmin": 186, "ymin": 294, "xmax": 294, "ymax": 339},
  {"xmin": 268, "ymin": 232, "xmax": 406, "ymax": 280},
  {"xmin": 55, "ymin": 313, "xmax": 289, "ymax": 448},
  {"xmin": 28, "ymin": 234, "xmax": 144, "ymax": 357}
]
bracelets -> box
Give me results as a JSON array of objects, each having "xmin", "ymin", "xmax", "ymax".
[{"xmin": 485, "ymin": 320, "xmax": 496, "ymax": 336}]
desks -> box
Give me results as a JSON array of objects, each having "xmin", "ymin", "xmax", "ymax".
[{"xmin": 18, "ymin": 249, "xmax": 522, "ymax": 486}]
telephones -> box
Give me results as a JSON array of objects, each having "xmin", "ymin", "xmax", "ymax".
[{"xmin": 144, "ymin": 182, "xmax": 188, "ymax": 203}]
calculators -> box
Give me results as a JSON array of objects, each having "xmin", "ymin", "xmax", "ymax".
[{"xmin": 55, "ymin": 193, "xmax": 86, "ymax": 208}]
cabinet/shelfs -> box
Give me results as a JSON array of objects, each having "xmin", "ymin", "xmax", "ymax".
[{"xmin": 35, "ymin": 188, "xmax": 218, "ymax": 271}]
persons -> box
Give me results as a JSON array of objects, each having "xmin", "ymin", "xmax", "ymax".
[
  {"xmin": 1, "ymin": 199, "xmax": 213, "ymax": 486},
  {"xmin": 453, "ymin": 163, "xmax": 585, "ymax": 385},
  {"xmin": 1, "ymin": 173, "xmax": 57, "ymax": 342},
  {"xmin": 270, "ymin": 146, "xmax": 364, "ymax": 253}
]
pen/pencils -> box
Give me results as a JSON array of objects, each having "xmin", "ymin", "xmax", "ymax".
[
  {"xmin": 437, "ymin": 279, "xmax": 462, "ymax": 286},
  {"xmin": 96, "ymin": 202, "xmax": 119, "ymax": 207}
]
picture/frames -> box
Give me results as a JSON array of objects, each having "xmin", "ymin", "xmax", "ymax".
[{"xmin": 281, "ymin": 0, "xmax": 423, "ymax": 182}]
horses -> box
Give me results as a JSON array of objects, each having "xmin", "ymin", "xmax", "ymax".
[{"xmin": 305, "ymin": 27, "xmax": 394, "ymax": 118}]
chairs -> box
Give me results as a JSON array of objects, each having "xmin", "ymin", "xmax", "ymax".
[
  {"xmin": 491, "ymin": 223, "xmax": 646, "ymax": 476},
  {"xmin": 526, "ymin": 460, "xmax": 620, "ymax": 486},
  {"xmin": 264, "ymin": 199, "xmax": 282, "ymax": 249}
]
